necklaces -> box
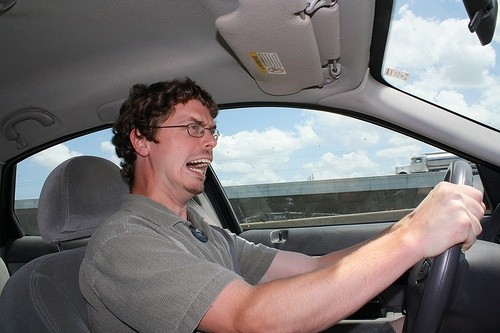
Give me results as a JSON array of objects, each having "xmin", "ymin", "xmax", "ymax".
[{"xmin": 189, "ymin": 221, "xmax": 208, "ymax": 243}]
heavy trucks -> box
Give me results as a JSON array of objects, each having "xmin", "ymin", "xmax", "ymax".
[{"xmin": 395, "ymin": 152, "xmax": 479, "ymax": 175}]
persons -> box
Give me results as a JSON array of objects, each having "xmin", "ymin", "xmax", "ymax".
[{"xmin": 79, "ymin": 76, "xmax": 487, "ymax": 333}]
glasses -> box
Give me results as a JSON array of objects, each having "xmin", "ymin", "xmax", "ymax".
[{"xmin": 140, "ymin": 122, "xmax": 220, "ymax": 141}]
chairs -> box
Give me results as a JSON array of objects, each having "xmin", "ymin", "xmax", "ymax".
[{"xmin": 0, "ymin": 156, "xmax": 130, "ymax": 333}]
